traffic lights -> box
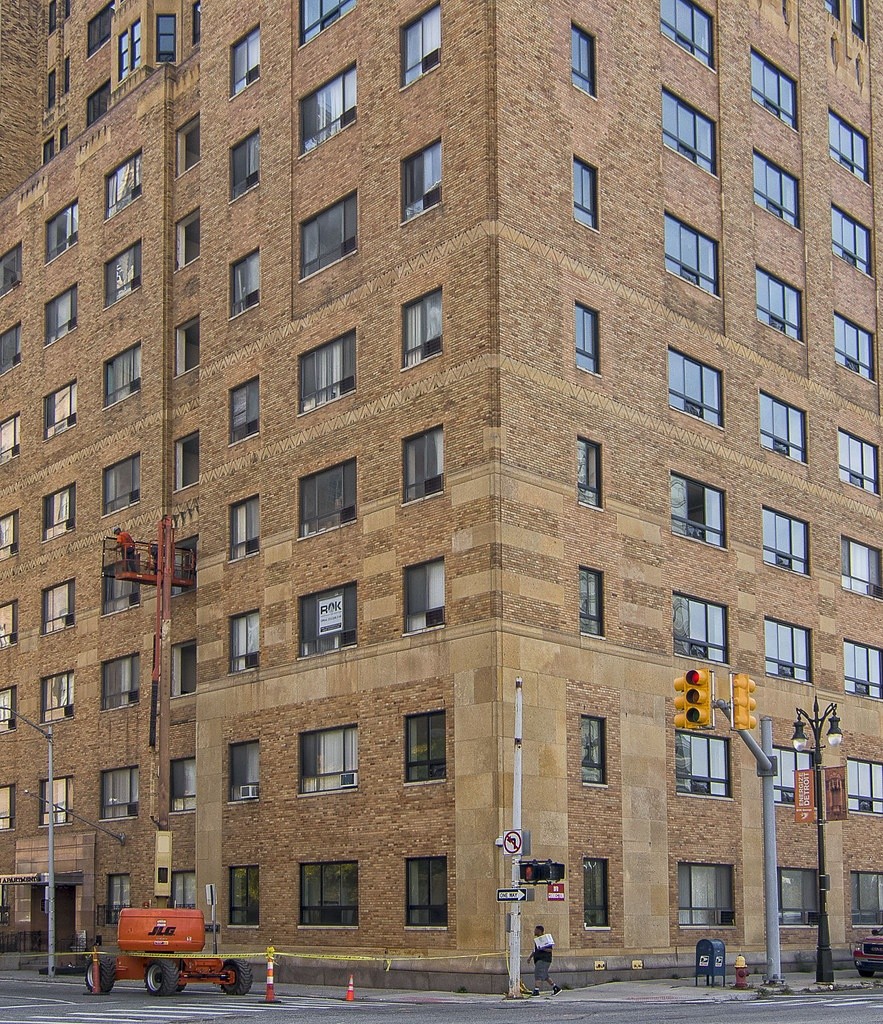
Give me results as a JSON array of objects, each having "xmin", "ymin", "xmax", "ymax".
[
  {"xmin": 520, "ymin": 863, "xmax": 551, "ymax": 881},
  {"xmin": 731, "ymin": 673, "xmax": 758, "ymax": 730},
  {"xmin": 684, "ymin": 670, "xmax": 711, "ymax": 728},
  {"xmin": 674, "ymin": 671, "xmax": 686, "ymax": 730}
]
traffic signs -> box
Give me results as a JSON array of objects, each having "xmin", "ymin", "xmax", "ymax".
[{"xmin": 495, "ymin": 888, "xmax": 528, "ymax": 903}]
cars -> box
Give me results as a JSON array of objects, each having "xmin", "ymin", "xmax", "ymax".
[{"xmin": 853, "ymin": 926, "xmax": 883, "ymax": 978}]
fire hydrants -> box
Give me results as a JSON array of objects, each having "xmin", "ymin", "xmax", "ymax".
[{"xmin": 734, "ymin": 954, "xmax": 750, "ymax": 990}]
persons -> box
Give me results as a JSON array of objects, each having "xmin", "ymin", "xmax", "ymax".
[
  {"xmin": 527, "ymin": 925, "xmax": 562, "ymax": 998},
  {"xmin": 110, "ymin": 527, "xmax": 136, "ymax": 571}
]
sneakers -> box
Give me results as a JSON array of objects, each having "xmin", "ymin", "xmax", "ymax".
[
  {"xmin": 530, "ymin": 990, "xmax": 540, "ymax": 997},
  {"xmin": 551, "ymin": 985, "xmax": 563, "ymax": 997}
]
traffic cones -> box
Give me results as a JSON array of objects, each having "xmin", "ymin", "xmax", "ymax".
[{"xmin": 345, "ymin": 973, "xmax": 355, "ymax": 1002}]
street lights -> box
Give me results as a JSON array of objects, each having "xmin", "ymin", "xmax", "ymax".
[{"xmin": 790, "ymin": 695, "xmax": 843, "ymax": 984}]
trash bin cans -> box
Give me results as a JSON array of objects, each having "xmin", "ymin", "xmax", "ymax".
[{"xmin": 696, "ymin": 938, "xmax": 725, "ymax": 988}]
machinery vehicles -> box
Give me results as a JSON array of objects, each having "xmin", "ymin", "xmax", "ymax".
[{"xmin": 88, "ymin": 517, "xmax": 255, "ymax": 994}]
